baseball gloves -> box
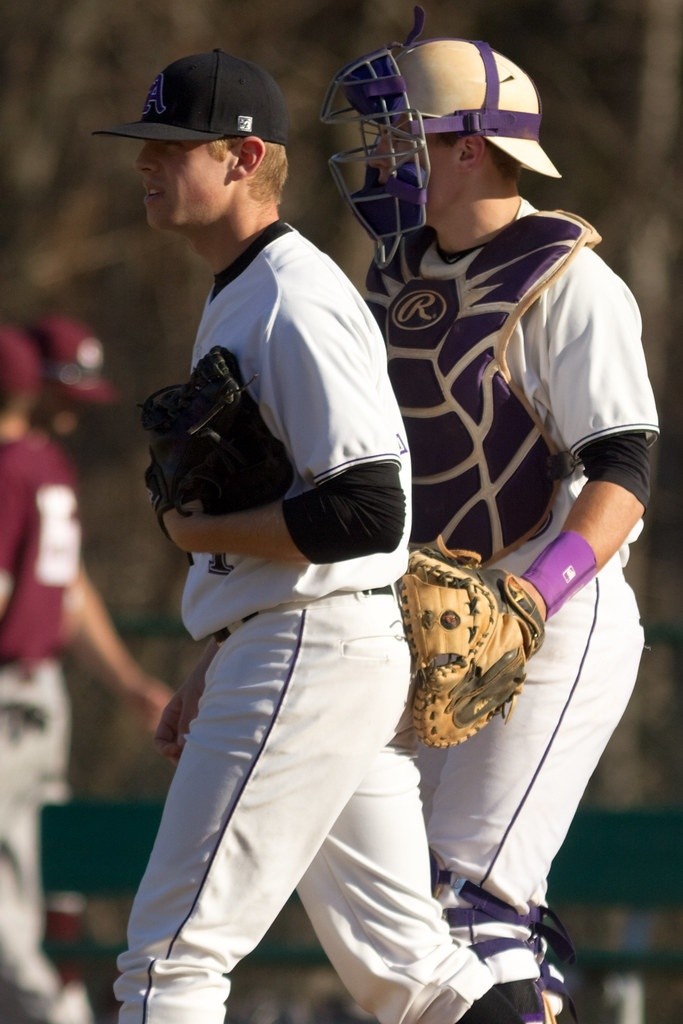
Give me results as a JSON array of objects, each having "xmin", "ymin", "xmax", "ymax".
[
  {"xmin": 395, "ymin": 532, "xmax": 551, "ymax": 752},
  {"xmin": 138, "ymin": 342, "xmax": 297, "ymax": 543}
]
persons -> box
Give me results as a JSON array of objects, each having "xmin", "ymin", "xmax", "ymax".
[
  {"xmin": 0, "ymin": 315, "xmax": 183, "ymax": 1024},
  {"xmin": 90, "ymin": 44, "xmax": 527, "ymax": 1023},
  {"xmin": 323, "ymin": 37, "xmax": 661, "ymax": 1023}
]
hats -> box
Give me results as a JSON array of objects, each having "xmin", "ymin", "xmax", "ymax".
[
  {"xmin": 0, "ymin": 313, "xmax": 116, "ymax": 403},
  {"xmin": 90, "ymin": 45, "xmax": 291, "ymax": 147}
]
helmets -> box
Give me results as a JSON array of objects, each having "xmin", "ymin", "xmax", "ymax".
[{"xmin": 397, "ymin": 41, "xmax": 563, "ymax": 179}]
